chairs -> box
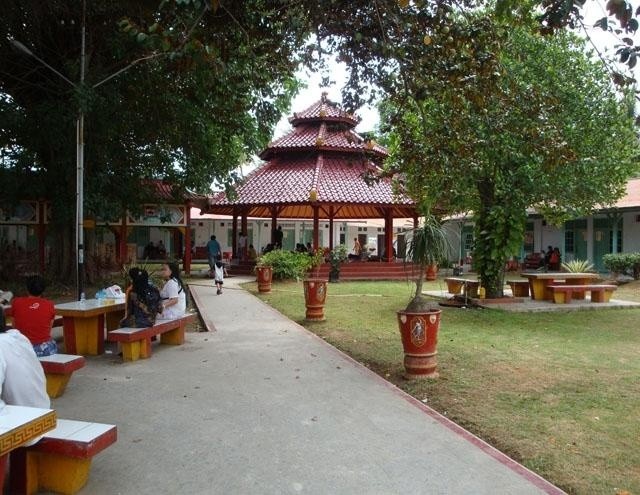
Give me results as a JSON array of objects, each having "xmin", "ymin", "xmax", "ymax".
[{"xmin": 223, "ymin": 246, "xmax": 376, "ymax": 262}]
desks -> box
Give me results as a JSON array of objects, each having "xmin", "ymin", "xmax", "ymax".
[
  {"xmin": 54, "ymin": 298, "xmax": 125, "ymax": 355},
  {"xmin": 0, "ymin": 404, "xmax": 57, "ymax": 457},
  {"xmin": 520, "ymin": 273, "xmax": 602, "ymax": 300}
]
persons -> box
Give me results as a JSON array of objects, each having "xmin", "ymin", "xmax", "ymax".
[
  {"xmin": 208, "ymin": 254, "xmax": 229, "ymax": 295},
  {"xmin": 13, "ymin": 274, "xmax": 59, "ymax": 357},
  {"xmin": 150, "ymin": 261, "xmax": 187, "ymax": 341},
  {"xmin": 549, "ymin": 247, "xmax": 560, "ymax": 263},
  {"xmin": 116, "ymin": 265, "xmax": 163, "ymax": 332},
  {"xmin": 0, "ymin": 296, "xmax": 54, "ymax": 481},
  {"xmin": 138, "ymin": 222, "xmax": 401, "ymax": 262},
  {"xmin": 537, "ymin": 249, "xmax": 549, "ymax": 267},
  {"xmin": 544, "ymin": 245, "xmax": 553, "ymax": 274}
]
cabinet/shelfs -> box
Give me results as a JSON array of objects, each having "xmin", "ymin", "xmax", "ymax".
[{"xmin": 195, "ymin": 247, "xmax": 208, "ymax": 259}]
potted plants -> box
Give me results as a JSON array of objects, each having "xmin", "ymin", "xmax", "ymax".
[
  {"xmin": 626, "ymin": 251, "xmax": 640, "ymax": 280},
  {"xmin": 602, "ymin": 254, "xmax": 634, "ymax": 281},
  {"xmin": 396, "ymin": 211, "xmax": 460, "ymax": 381}
]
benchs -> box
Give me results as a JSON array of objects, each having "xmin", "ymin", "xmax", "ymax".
[
  {"xmin": 108, "ymin": 313, "xmax": 197, "ymax": 361},
  {"xmin": 5, "ymin": 315, "xmax": 63, "ymax": 331},
  {"xmin": 506, "ymin": 280, "xmax": 566, "ymax": 297},
  {"xmin": 546, "ymin": 285, "xmax": 617, "ymax": 303},
  {"xmin": 444, "ymin": 278, "xmax": 481, "ymax": 298},
  {"xmin": 37, "ymin": 353, "xmax": 85, "ymax": 398},
  {"xmin": 143, "ymin": 247, "xmax": 166, "ymax": 259},
  {"xmin": 9, "ymin": 419, "xmax": 117, "ymax": 495}
]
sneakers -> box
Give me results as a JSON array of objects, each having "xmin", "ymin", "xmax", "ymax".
[{"xmin": 217, "ymin": 289, "xmax": 223, "ymax": 295}]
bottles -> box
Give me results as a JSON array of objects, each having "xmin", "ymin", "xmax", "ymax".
[
  {"xmin": 95, "ymin": 291, "xmax": 100, "ymax": 307},
  {"xmin": 79, "ymin": 292, "xmax": 87, "ymax": 309}
]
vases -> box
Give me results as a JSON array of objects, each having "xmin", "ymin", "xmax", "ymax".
[
  {"xmin": 256, "ymin": 266, "xmax": 272, "ymax": 292},
  {"xmin": 303, "ymin": 279, "xmax": 326, "ymax": 322}
]
